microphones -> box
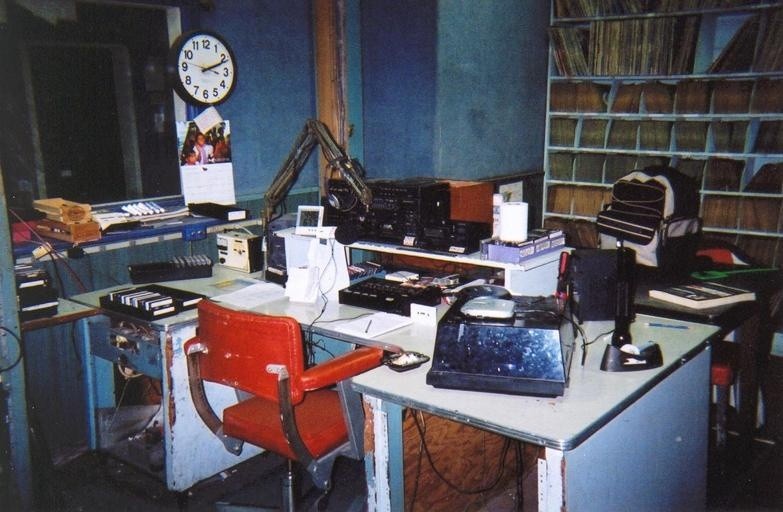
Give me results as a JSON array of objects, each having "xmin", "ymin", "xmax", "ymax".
[{"xmin": 334, "ymin": 215, "xmax": 370, "ymax": 245}]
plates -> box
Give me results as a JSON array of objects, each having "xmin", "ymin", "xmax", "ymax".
[{"xmin": 381, "ymin": 352, "xmax": 431, "ymax": 372}]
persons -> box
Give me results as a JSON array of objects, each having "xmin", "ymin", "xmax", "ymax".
[{"xmin": 178, "ymin": 121, "xmax": 232, "ymax": 166}]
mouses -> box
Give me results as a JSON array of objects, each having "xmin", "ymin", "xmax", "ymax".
[
  {"xmin": 388, "ymin": 350, "xmax": 431, "ymax": 371},
  {"xmin": 460, "ymin": 295, "xmax": 518, "ymax": 321}
]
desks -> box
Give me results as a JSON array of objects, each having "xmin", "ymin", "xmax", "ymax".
[
  {"xmin": 65, "ymin": 261, "xmax": 723, "ymax": 509},
  {"xmin": 342, "ymin": 219, "xmax": 576, "ymax": 298},
  {"xmin": 627, "ymin": 271, "xmax": 767, "ymax": 459}
]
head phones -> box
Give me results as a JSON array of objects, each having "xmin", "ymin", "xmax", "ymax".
[{"xmin": 323, "ymin": 156, "xmax": 367, "ymax": 213}]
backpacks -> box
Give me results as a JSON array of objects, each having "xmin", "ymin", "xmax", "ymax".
[{"xmin": 595, "ymin": 166, "xmax": 702, "ymax": 282}]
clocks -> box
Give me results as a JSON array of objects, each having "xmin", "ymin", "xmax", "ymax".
[{"xmin": 166, "ymin": 28, "xmax": 237, "ymax": 108}]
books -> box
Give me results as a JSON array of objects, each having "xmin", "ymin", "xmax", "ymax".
[
  {"xmin": 124, "ymin": 202, "xmax": 246, "ymax": 229},
  {"xmin": 543, "ymin": 78, "xmax": 783, "ymax": 268},
  {"xmin": 546, "ymin": 13, "xmax": 702, "ymax": 77},
  {"xmin": 706, "ymin": 8, "xmax": 783, "ymax": 74},
  {"xmin": 647, "ymin": 280, "xmax": 756, "ymax": 311},
  {"xmin": 553, "ymin": 1, "xmax": 783, "ymax": 18}
]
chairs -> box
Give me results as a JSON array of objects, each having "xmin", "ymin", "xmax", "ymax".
[{"xmin": 179, "ymin": 297, "xmax": 385, "ymax": 511}]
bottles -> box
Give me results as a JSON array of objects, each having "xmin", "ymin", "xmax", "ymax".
[{"xmin": 491, "ymin": 193, "xmax": 504, "ymax": 238}]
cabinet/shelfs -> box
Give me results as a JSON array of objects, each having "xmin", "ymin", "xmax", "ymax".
[{"xmin": 538, "ymin": 1, "xmax": 781, "ymax": 322}]
